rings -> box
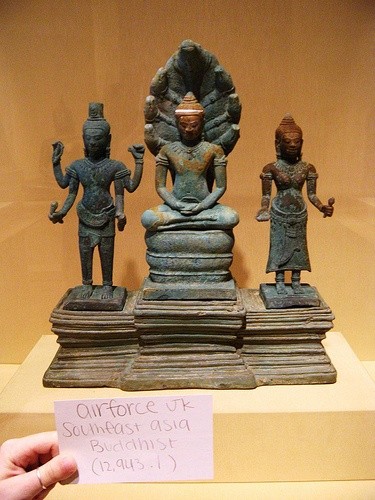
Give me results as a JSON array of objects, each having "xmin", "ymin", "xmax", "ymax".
[{"xmin": 35, "ymin": 467, "xmax": 48, "ymax": 490}]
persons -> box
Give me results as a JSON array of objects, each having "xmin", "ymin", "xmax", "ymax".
[
  {"xmin": 48, "ymin": 101, "xmax": 146, "ymax": 299},
  {"xmin": 255, "ymin": 114, "xmax": 333, "ymax": 296},
  {"xmin": 142, "ymin": 91, "xmax": 240, "ymax": 232},
  {"xmin": 0, "ymin": 430, "xmax": 76, "ymax": 500}
]
